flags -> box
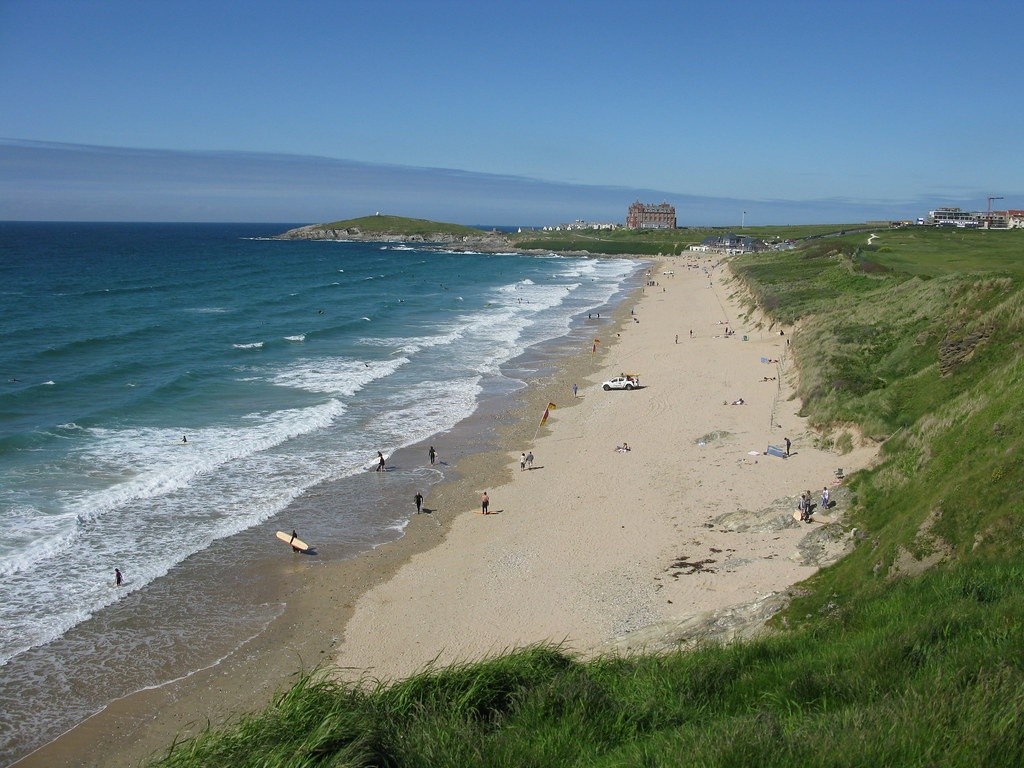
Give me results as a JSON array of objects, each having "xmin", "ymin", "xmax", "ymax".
[{"xmin": 540, "ymin": 402, "xmax": 557, "ymax": 427}]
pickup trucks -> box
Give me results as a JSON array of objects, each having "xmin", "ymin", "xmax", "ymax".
[{"xmin": 602, "ymin": 376, "xmax": 638, "ymax": 391}]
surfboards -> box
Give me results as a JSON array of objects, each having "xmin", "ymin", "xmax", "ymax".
[{"xmin": 276, "ymin": 531, "xmax": 309, "ymax": 551}]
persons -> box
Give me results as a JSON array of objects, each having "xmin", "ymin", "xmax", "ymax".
[
  {"xmin": 377, "ymin": 451, "xmax": 382, "ymax": 465},
  {"xmin": 289, "ymin": 530, "xmax": 298, "ymax": 553},
  {"xmin": 182, "ymin": 436, "xmax": 187, "ymax": 443},
  {"xmin": 414, "ymin": 492, "xmax": 424, "ymax": 515},
  {"xmin": 429, "ymin": 446, "xmax": 435, "ymax": 465},
  {"xmin": 314, "ymin": 238, "xmax": 796, "ymax": 386},
  {"xmin": 616, "ymin": 443, "xmax": 627, "ymax": 452},
  {"xmin": 379, "ymin": 455, "xmax": 386, "ymax": 472},
  {"xmin": 822, "ymin": 487, "xmax": 829, "ymax": 509},
  {"xmin": 482, "ymin": 492, "xmax": 489, "ymax": 515},
  {"xmin": 573, "ymin": 384, "xmax": 578, "ymax": 397},
  {"xmin": 800, "ymin": 490, "xmax": 813, "ymax": 521},
  {"xmin": 526, "ymin": 451, "xmax": 534, "ymax": 469},
  {"xmin": 784, "ymin": 438, "xmax": 791, "ymax": 456},
  {"xmin": 733, "ymin": 398, "xmax": 744, "ymax": 405},
  {"xmin": 520, "ymin": 453, "xmax": 527, "ymax": 471},
  {"xmin": 114, "ymin": 568, "xmax": 122, "ymax": 587}
]
